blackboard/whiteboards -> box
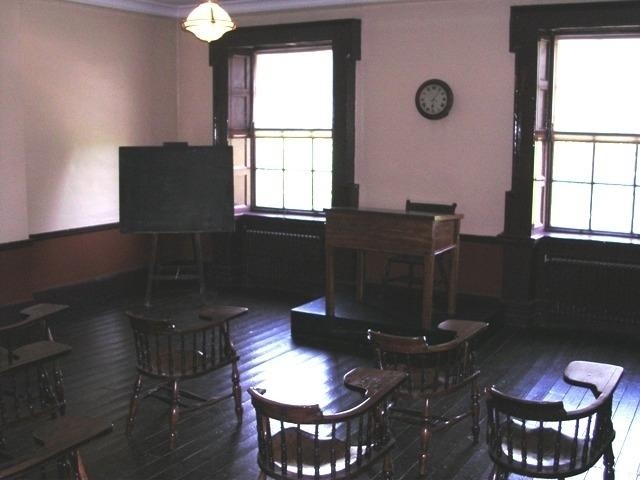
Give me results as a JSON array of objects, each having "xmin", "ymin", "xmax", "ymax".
[{"xmin": 119, "ymin": 145, "xmax": 235, "ymax": 235}]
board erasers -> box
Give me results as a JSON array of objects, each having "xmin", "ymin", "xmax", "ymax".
[{"xmin": 164, "ymin": 141, "xmax": 188, "ymax": 146}]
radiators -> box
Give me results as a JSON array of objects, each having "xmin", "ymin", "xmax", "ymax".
[
  {"xmin": 538, "ymin": 253, "xmax": 640, "ymax": 331},
  {"xmin": 239, "ymin": 223, "xmax": 322, "ymax": 299}
]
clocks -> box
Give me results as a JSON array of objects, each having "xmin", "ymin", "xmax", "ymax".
[{"xmin": 415, "ymin": 78, "xmax": 453, "ymax": 119}]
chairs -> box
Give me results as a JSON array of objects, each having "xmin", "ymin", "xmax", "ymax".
[
  {"xmin": 124, "ymin": 304, "xmax": 251, "ymax": 450},
  {"xmin": 246, "ymin": 314, "xmax": 489, "ymax": 479},
  {"xmin": 482, "ymin": 355, "xmax": 626, "ymax": 480},
  {"xmin": 1, "ymin": 300, "xmax": 117, "ymax": 480},
  {"xmin": 379, "ymin": 200, "xmax": 459, "ymax": 329}
]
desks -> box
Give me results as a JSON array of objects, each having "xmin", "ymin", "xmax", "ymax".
[{"xmin": 321, "ymin": 205, "xmax": 467, "ymax": 337}]
